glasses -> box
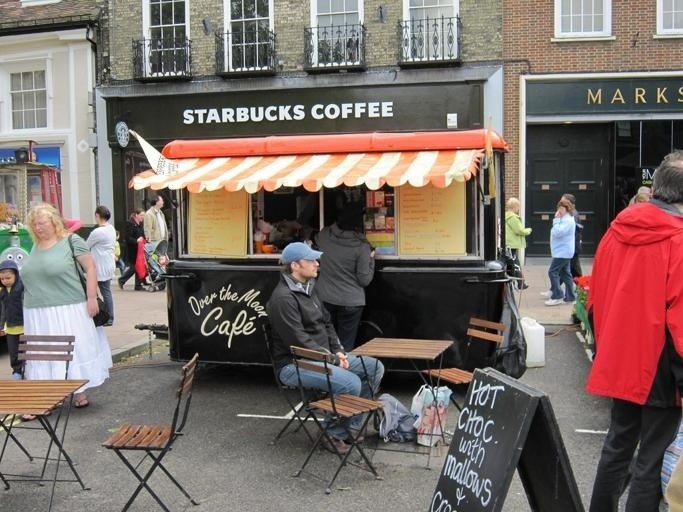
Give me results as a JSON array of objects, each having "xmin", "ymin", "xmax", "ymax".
[{"xmin": 31, "ymin": 220, "xmax": 53, "ymax": 229}]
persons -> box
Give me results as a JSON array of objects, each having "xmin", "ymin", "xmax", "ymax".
[
  {"xmin": 544, "ymin": 198, "xmax": 577, "ymax": 306},
  {"xmin": 84, "ymin": 206, "xmax": 117, "ymax": 326},
  {"xmin": 153, "ymin": 251, "xmax": 174, "ymax": 281},
  {"xmin": 628, "ymin": 186, "xmax": 654, "ymax": 204},
  {"xmin": 505, "ymin": 196, "xmax": 533, "ymax": 291},
  {"xmin": 114, "ymin": 229, "xmax": 128, "ymax": 286},
  {"xmin": 311, "ymin": 200, "xmax": 376, "ymax": 354},
  {"xmin": 19, "ymin": 202, "xmax": 113, "ymax": 421},
  {"xmin": 538, "ymin": 194, "xmax": 585, "ymax": 299},
  {"xmin": 0, "ymin": 258, "xmax": 26, "ymax": 381},
  {"xmin": 1, "ymin": 204, "xmax": 26, "ymax": 232},
  {"xmin": 584, "ymin": 150, "xmax": 681, "ymax": 511},
  {"xmin": 265, "ymin": 242, "xmax": 384, "ymax": 453},
  {"xmin": 117, "ymin": 206, "xmax": 151, "ymax": 292},
  {"xmin": 140, "ymin": 195, "xmax": 170, "ymax": 286}
]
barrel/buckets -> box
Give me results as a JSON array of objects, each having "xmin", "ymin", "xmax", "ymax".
[{"xmin": 521, "ymin": 315, "xmax": 545, "ymax": 369}]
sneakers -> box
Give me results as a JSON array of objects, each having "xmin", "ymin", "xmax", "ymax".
[
  {"xmin": 544, "ymin": 298, "xmax": 563, "ymax": 306},
  {"xmin": 347, "ymin": 435, "xmax": 365, "ymax": 444},
  {"xmin": 118, "ymin": 278, "xmax": 124, "ymax": 290},
  {"xmin": 9, "ymin": 373, "xmax": 22, "ymax": 380},
  {"xmin": 541, "ymin": 290, "xmax": 553, "ymax": 297},
  {"xmin": 134, "ymin": 285, "xmax": 147, "ymax": 291},
  {"xmin": 317, "ymin": 426, "xmax": 349, "ymax": 455},
  {"xmin": 517, "ymin": 284, "xmax": 530, "ymax": 290},
  {"xmin": 102, "ymin": 321, "xmax": 113, "ymax": 327},
  {"xmin": 563, "ymin": 300, "xmax": 577, "ymax": 304}
]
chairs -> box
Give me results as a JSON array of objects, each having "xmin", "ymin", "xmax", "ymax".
[
  {"xmin": 287, "ymin": 344, "xmax": 383, "ymax": 496},
  {"xmin": 425, "ymin": 318, "xmax": 505, "ymax": 444},
  {"xmin": 102, "ymin": 353, "xmax": 199, "ymax": 510},
  {"xmin": 260, "ymin": 322, "xmax": 328, "ymax": 447},
  {"xmin": 4, "ymin": 334, "xmax": 75, "ymax": 471}
]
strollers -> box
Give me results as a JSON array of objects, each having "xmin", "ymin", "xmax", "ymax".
[{"xmin": 143, "ymin": 239, "xmax": 167, "ymax": 290}]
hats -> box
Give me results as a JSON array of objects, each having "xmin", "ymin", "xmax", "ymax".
[{"xmin": 278, "ymin": 242, "xmax": 323, "ymax": 266}]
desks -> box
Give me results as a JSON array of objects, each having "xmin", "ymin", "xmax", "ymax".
[
  {"xmin": 0, "ymin": 378, "xmax": 88, "ymax": 512},
  {"xmin": 341, "ymin": 338, "xmax": 454, "ymax": 471}
]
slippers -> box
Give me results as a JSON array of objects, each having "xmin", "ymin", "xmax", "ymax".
[
  {"xmin": 72, "ymin": 396, "xmax": 88, "ymax": 409},
  {"xmin": 21, "ymin": 415, "xmax": 37, "ymax": 420}
]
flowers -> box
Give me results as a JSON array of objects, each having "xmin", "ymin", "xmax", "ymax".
[{"xmin": 573, "ymin": 276, "xmax": 591, "ymax": 305}]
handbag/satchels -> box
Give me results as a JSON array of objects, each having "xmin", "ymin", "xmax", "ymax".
[{"xmin": 67, "ymin": 233, "xmax": 108, "ymax": 326}]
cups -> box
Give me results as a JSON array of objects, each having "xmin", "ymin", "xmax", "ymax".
[{"xmin": 261, "ymin": 245, "xmax": 278, "ymax": 252}]
